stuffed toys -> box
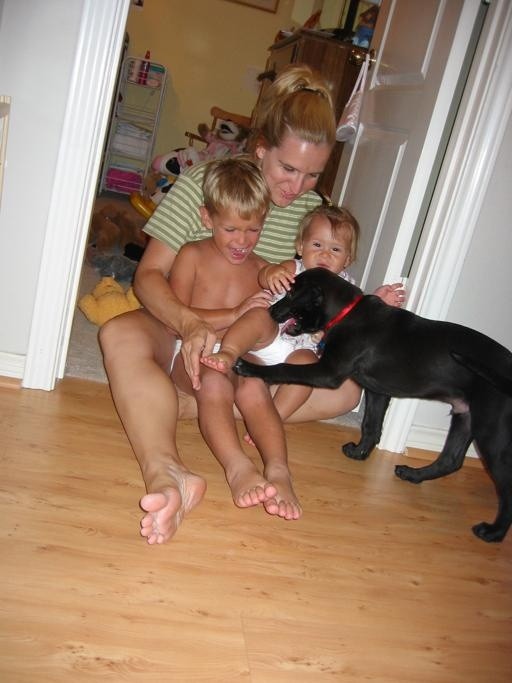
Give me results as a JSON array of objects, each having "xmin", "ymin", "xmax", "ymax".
[
  {"xmin": 76, "ymin": 274, "xmax": 142, "ymax": 328},
  {"xmin": 83, "ymin": 202, "xmax": 142, "ymax": 267},
  {"xmin": 124, "ymin": 242, "xmax": 147, "ymax": 262},
  {"xmin": 175, "ymin": 117, "xmax": 251, "ymax": 170},
  {"xmin": 95, "ymin": 254, "xmax": 140, "ymax": 284},
  {"xmin": 148, "ymin": 147, "xmax": 187, "ymax": 206}
]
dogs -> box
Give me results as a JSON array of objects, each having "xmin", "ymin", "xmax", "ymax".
[{"xmin": 233, "ymin": 267, "xmax": 512, "ymax": 543}]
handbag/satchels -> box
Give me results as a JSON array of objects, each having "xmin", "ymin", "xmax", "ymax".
[{"xmin": 334, "ymin": 53, "xmax": 370, "ymax": 142}]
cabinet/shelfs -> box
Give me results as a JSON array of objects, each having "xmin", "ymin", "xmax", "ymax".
[
  {"xmin": 249, "ymin": 34, "xmax": 363, "ymax": 212},
  {"xmin": 99, "ymin": 55, "xmax": 169, "ymax": 203}
]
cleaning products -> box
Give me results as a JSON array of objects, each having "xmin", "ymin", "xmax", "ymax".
[
  {"xmin": 138, "ymin": 49, "xmax": 151, "ymax": 85},
  {"xmin": 128, "ymin": 60, "xmax": 142, "ymax": 82}
]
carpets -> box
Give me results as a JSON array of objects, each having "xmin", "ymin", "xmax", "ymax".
[{"xmin": 62, "ymin": 247, "xmax": 274, "ymax": 403}]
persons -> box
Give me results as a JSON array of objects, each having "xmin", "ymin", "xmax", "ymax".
[
  {"xmin": 94, "ymin": 59, "xmax": 362, "ymax": 547},
  {"xmin": 196, "ymin": 203, "xmax": 409, "ymax": 450},
  {"xmin": 163, "ymin": 151, "xmax": 305, "ymax": 521}
]
